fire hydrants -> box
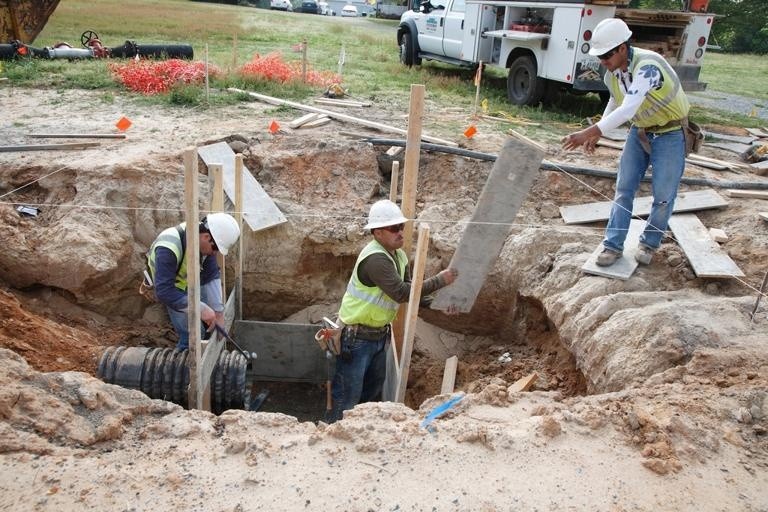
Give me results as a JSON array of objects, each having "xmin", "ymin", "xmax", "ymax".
[{"xmin": 86, "ymin": 36, "xmax": 110, "ymax": 61}]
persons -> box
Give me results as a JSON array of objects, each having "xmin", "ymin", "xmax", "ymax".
[
  {"xmin": 147, "ymin": 211, "xmax": 240, "ymax": 349},
  {"xmin": 321, "ymin": 198, "xmax": 460, "ymax": 423},
  {"xmin": 559, "ymin": 17, "xmax": 692, "ymax": 268}
]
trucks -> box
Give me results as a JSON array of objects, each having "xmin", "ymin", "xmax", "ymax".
[{"xmin": 395, "ymin": 0, "xmax": 728, "ymax": 111}]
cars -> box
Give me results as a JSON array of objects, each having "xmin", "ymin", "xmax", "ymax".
[{"xmin": 269, "ymin": 0, "xmax": 360, "ymax": 17}]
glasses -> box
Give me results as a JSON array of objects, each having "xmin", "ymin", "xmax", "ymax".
[
  {"xmin": 597, "ymin": 50, "xmax": 614, "ymax": 59},
  {"xmin": 384, "ymin": 223, "xmax": 404, "ymax": 233}
]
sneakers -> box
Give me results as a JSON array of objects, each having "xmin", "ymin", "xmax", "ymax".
[
  {"xmin": 635, "ymin": 245, "xmax": 654, "ymax": 264},
  {"xmin": 596, "ymin": 248, "xmax": 623, "ymax": 266}
]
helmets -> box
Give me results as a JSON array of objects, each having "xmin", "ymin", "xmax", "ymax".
[
  {"xmin": 207, "ymin": 213, "xmax": 240, "ymax": 256},
  {"xmin": 589, "ymin": 18, "xmax": 633, "ymax": 56},
  {"xmin": 363, "ymin": 199, "xmax": 408, "ymax": 229}
]
